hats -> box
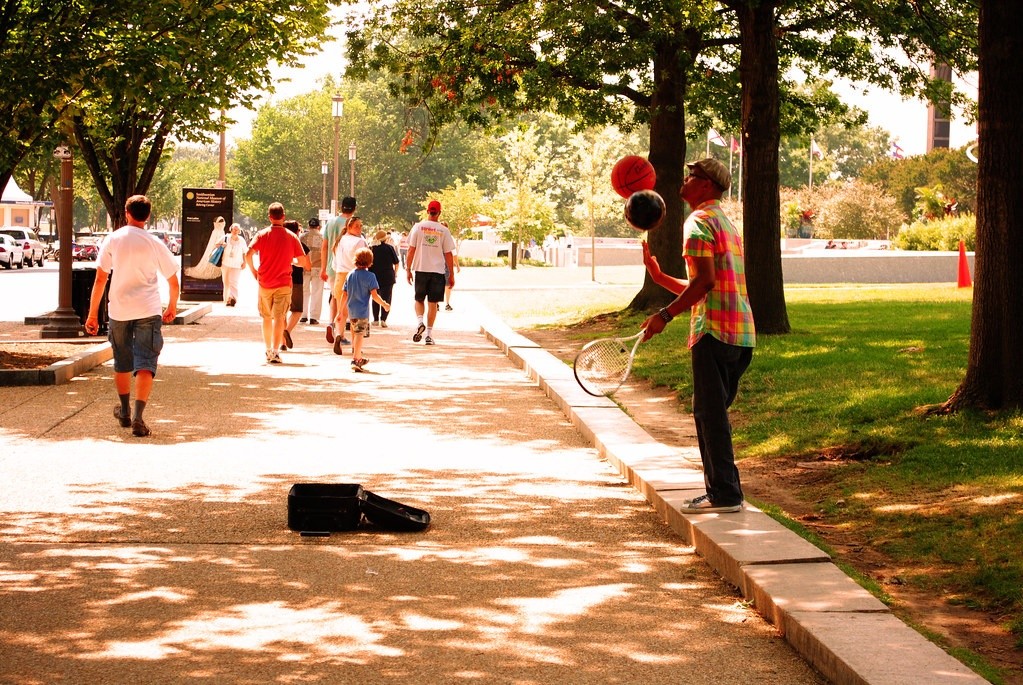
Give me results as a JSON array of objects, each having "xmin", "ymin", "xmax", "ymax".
[
  {"xmin": 342, "ymin": 197, "xmax": 356, "ymax": 210},
  {"xmin": 428, "ymin": 201, "xmax": 441, "ymax": 213},
  {"xmin": 687, "ymin": 158, "xmax": 731, "ymax": 190},
  {"xmin": 308, "ymin": 218, "xmax": 319, "ymax": 227}
]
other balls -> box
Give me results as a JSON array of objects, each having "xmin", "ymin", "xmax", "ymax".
[{"xmin": 624, "ymin": 189, "xmax": 665, "ymax": 231}]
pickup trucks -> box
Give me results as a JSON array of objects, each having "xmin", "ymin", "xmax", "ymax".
[{"xmin": 455, "ymin": 228, "xmax": 530, "ymax": 260}]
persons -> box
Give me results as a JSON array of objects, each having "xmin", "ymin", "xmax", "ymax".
[
  {"xmin": 642, "ymin": 158, "xmax": 757, "ymax": 514},
  {"xmin": 405, "ymin": 200, "xmax": 455, "ymax": 345},
  {"xmin": 84, "ymin": 195, "xmax": 179, "ymax": 438},
  {"xmin": 437, "ymin": 222, "xmax": 461, "ymax": 311},
  {"xmin": 332, "ymin": 217, "xmax": 367, "ymax": 353},
  {"xmin": 321, "ymin": 197, "xmax": 356, "ymax": 345},
  {"xmin": 215, "ymin": 223, "xmax": 248, "ymax": 307},
  {"xmin": 335, "ymin": 248, "xmax": 391, "ymax": 373},
  {"xmin": 825, "ymin": 241, "xmax": 835, "ymax": 249},
  {"xmin": 386, "ymin": 228, "xmax": 409, "ymax": 269},
  {"xmin": 299, "ymin": 218, "xmax": 324, "ymax": 324},
  {"xmin": 368, "ymin": 230, "xmax": 400, "ymax": 328},
  {"xmin": 841, "ymin": 242, "xmax": 847, "ymax": 249},
  {"xmin": 280, "ymin": 220, "xmax": 312, "ymax": 350},
  {"xmin": 245, "ymin": 202, "xmax": 306, "ymax": 363}
]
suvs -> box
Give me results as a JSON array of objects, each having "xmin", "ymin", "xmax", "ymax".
[{"xmin": 0, "ymin": 227, "xmax": 44, "ymax": 267}]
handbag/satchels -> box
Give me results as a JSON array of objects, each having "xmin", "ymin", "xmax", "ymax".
[{"xmin": 209, "ymin": 236, "xmax": 227, "ymax": 267}]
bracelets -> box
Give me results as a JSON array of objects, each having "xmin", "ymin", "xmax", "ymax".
[
  {"xmin": 659, "ymin": 307, "xmax": 673, "ymax": 322},
  {"xmin": 88, "ymin": 316, "xmax": 97, "ymax": 318}
]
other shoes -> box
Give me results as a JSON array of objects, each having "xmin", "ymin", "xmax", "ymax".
[
  {"xmin": 359, "ymin": 358, "xmax": 370, "ymax": 366},
  {"xmin": 132, "ymin": 420, "xmax": 151, "ymax": 436},
  {"xmin": 299, "ymin": 318, "xmax": 308, "ymax": 322},
  {"xmin": 226, "ymin": 299, "xmax": 237, "ymax": 307},
  {"xmin": 363, "ymin": 324, "xmax": 370, "ymax": 337},
  {"xmin": 113, "ymin": 404, "xmax": 132, "ymax": 427},
  {"xmin": 380, "ymin": 321, "xmax": 387, "ymax": 327},
  {"xmin": 372, "ymin": 321, "xmax": 379, "ymax": 325},
  {"xmin": 445, "ymin": 304, "xmax": 452, "ymax": 311},
  {"xmin": 350, "ymin": 361, "xmax": 363, "ymax": 372},
  {"xmin": 437, "ymin": 305, "xmax": 439, "ymax": 311},
  {"xmin": 310, "ymin": 319, "xmax": 319, "ymax": 323},
  {"xmin": 266, "ymin": 331, "xmax": 293, "ymax": 363},
  {"xmin": 326, "ymin": 325, "xmax": 351, "ymax": 356}
]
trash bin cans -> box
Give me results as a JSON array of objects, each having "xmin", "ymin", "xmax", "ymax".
[{"xmin": 73, "ymin": 269, "xmax": 113, "ymax": 336}]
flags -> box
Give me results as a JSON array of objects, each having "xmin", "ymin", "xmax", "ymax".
[
  {"xmin": 812, "ymin": 140, "xmax": 823, "ymax": 160},
  {"xmin": 731, "ymin": 138, "xmax": 740, "ymax": 154},
  {"xmin": 892, "ymin": 142, "xmax": 904, "ymax": 159},
  {"xmin": 708, "ymin": 128, "xmax": 727, "ymax": 147}
]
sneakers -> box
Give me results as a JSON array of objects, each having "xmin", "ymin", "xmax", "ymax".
[
  {"xmin": 680, "ymin": 493, "xmax": 743, "ymax": 512},
  {"xmin": 413, "ymin": 323, "xmax": 425, "ymax": 343},
  {"xmin": 426, "ymin": 336, "xmax": 435, "ymax": 346}
]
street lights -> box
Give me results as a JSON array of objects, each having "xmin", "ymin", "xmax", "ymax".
[
  {"xmin": 330, "ymin": 91, "xmax": 345, "ymax": 217},
  {"xmin": 348, "ymin": 143, "xmax": 356, "ymax": 198},
  {"xmin": 320, "ymin": 157, "xmax": 328, "ymax": 238}
]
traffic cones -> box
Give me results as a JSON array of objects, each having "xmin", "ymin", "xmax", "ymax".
[{"xmin": 958, "ymin": 240, "xmax": 972, "ymax": 288}]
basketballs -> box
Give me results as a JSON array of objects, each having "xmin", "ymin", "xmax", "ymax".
[{"xmin": 611, "ymin": 155, "xmax": 655, "ymax": 199}]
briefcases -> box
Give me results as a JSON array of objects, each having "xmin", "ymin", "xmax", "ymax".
[{"xmin": 287, "ymin": 483, "xmax": 430, "ymax": 533}]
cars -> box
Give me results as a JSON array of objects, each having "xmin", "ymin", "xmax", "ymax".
[
  {"xmin": 0, "ymin": 234, "xmax": 25, "ymax": 269},
  {"xmin": 72, "ymin": 231, "xmax": 182, "ymax": 261}
]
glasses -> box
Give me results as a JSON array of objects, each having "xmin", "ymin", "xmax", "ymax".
[
  {"xmin": 686, "ymin": 172, "xmax": 709, "ymax": 183},
  {"xmin": 348, "ymin": 216, "xmax": 361, "ymax": 225}
]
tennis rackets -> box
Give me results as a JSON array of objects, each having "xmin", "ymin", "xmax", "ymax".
[{"xmin": 573, "ymin": 326, "xmax": 647, "ymax": 398}]
